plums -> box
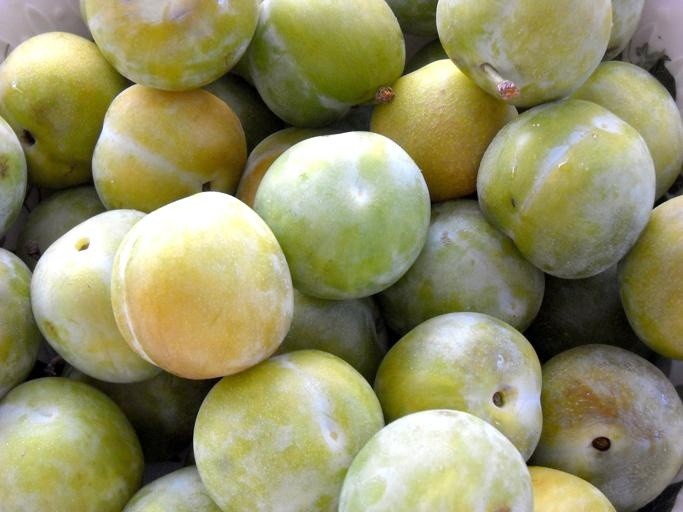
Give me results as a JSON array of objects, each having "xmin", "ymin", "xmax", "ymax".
[{"xmin": 1, "ymin": 1, "xmax": 683, "ymax": 511}]
